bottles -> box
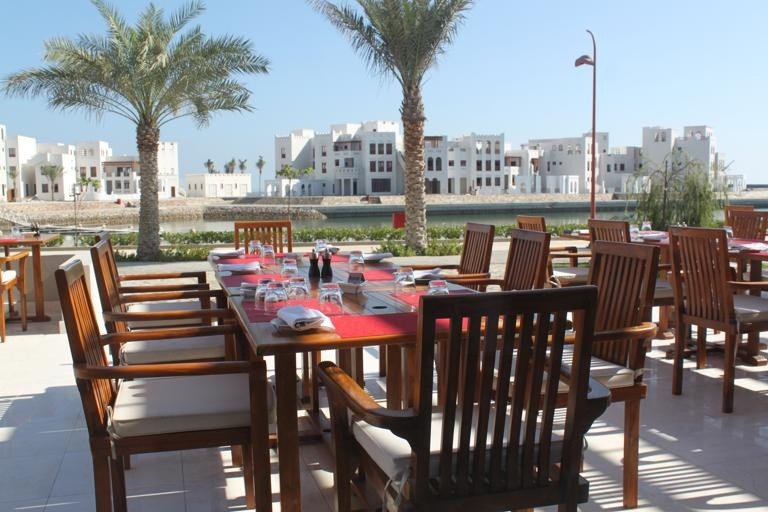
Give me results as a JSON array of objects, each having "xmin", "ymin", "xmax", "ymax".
[
  {"xmin": 629, "ymin": 219, "xmax": 652, "ymax": 239},
  {"xmin": 308, "ymin": 248, "xmax": 319, "ymax": 291},
  {"xmin": 321, "ymin": 248, "xmax": 333, "ymax": 284}
]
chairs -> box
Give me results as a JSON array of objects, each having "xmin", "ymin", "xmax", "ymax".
[
  {"xmin": 318, "ymin": 284, "xmax": 612, "ymax": 512},
  {"xmin": 379, "ymin": 204, "xmax": 768, "ymax": 415},
  {"xmin": 0, "ymin": 229, "xmax": 59, "ymax": 344},
  {"xmin": 233, "ymin": 220, "xmax": 294, "ymax": 254},
  {"xmin": 55, "ymin": 226, "xmax": 276, "ymax": 512}
]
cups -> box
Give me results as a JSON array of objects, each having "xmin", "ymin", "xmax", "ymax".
[
  {"xmin": 425, "ymin": 280, "xmax": 450, "ymax": 297},
  {"xmin": 394, "ymin": 268, "xmax": 416, "ymax": 298},
  {"xmin": 721, "ymin": 226, "xmax": 734, "ymax": 249},
  {"xmin": 349, "ymin": 251, "xmax": 364, "ymax": 273},
  {"xmin": 315, "ymin": 239, "xmax": 328, "ymax": 255},
  {"xmin": 318, "ymin": 282, "xmax": 344, "ymax": 319},
  {"xmin": 240, "ymin": 239, "xmax": 308, "ymax": 315}
]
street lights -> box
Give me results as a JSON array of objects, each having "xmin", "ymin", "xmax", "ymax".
[{"xmin": 574, "ymin": 53, "xmax": 600, "ymax": 232}]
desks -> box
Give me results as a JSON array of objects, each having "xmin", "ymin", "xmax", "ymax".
[{"xmin": 207, "ymin": 250, "xmax": 573, "ymax": 512}]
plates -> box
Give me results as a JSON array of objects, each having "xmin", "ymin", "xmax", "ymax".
[{"xmin": 638, "ymin": 234, "xmax": 667, "ymax": 242}]
